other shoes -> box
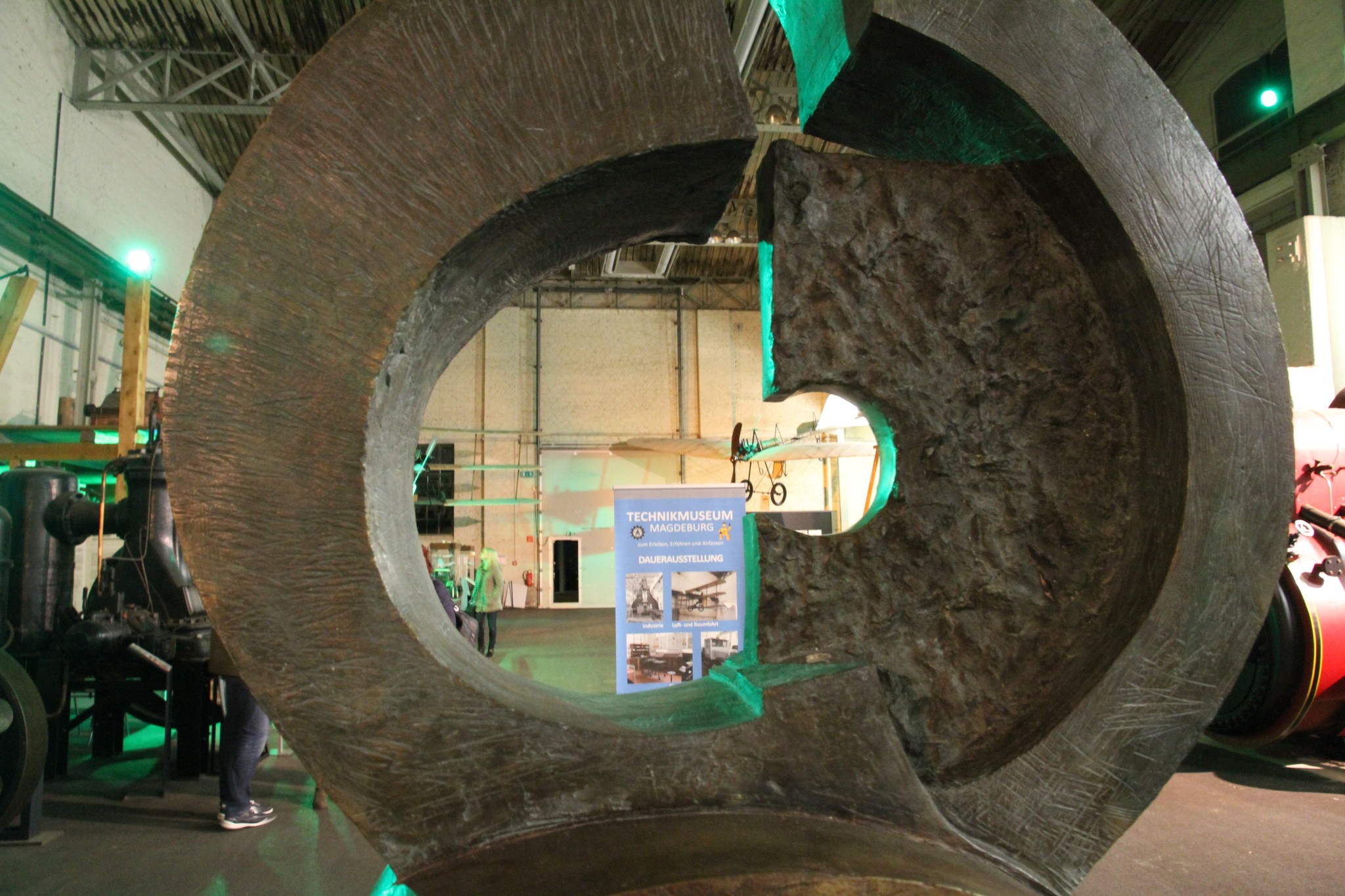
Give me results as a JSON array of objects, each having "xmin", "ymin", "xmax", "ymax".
[{"xmin": 484, "ymin": 649, "xmax": 494, "ymax": 658}]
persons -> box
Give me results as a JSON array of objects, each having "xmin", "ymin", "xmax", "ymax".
[
  {"xmin": 469, "ymin": 548, "xmax": 504, "ymax": 657},
  {"xmin": 422, "ymin": 544, "xmax": 456, "ymax": 628},
  {"xmin": 84, "ymin": 486, "xmax": 276, "ymax": 829}
]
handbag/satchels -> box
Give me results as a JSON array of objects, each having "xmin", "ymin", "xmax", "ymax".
[{"xmin": 454, "ymin": 603, "xmax": 479, "ymax": 647}]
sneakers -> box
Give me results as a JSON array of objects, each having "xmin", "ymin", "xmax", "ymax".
[{"xmin": 215, "ymin": 798, "xmax": 277, "ymax": 829}]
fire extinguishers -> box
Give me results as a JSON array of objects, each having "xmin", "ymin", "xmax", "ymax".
[{"xmin": 523, "ymin": 571, "xmax": 533, "ymax": 586}]
